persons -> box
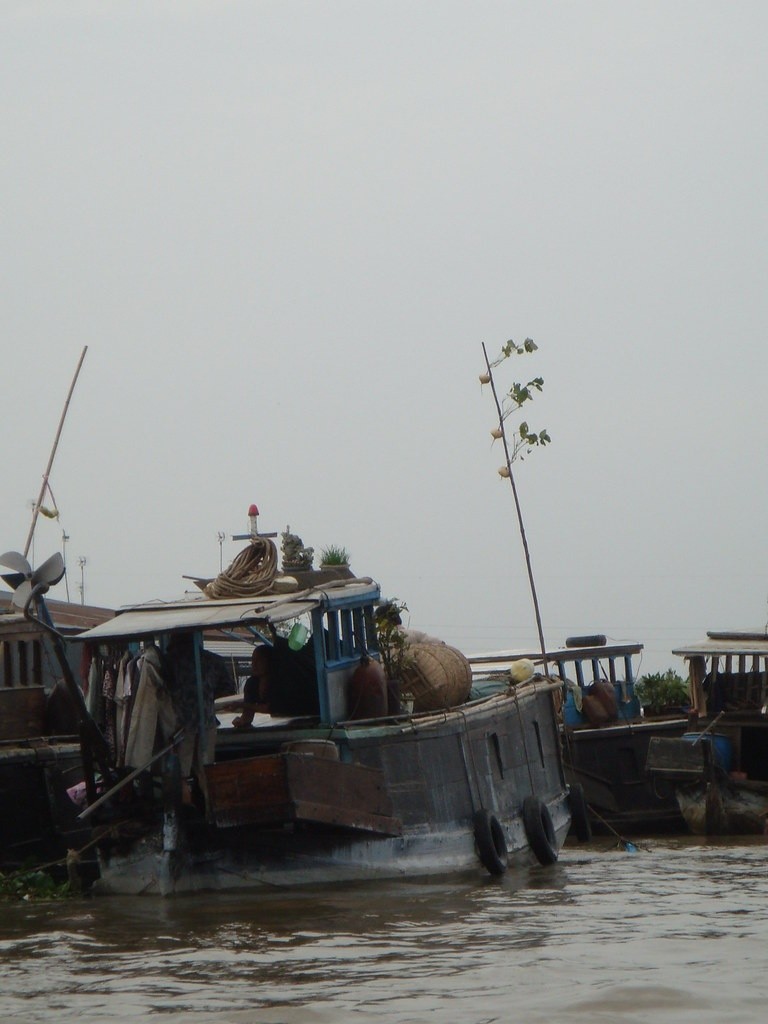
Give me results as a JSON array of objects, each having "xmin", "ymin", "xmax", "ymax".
[{"xmin": 228, "ymin": 645, "xmax": 311, "ymax": 729}]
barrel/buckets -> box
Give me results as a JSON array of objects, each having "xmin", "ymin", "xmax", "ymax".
[{"xmin": 683, "ymin": 733, "xmax": 732, "ymax": 772}]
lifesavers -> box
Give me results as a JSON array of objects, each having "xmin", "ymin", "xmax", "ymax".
[
  {"xmin": 474, "ymin": 808, "xmax": 511, "ymax": 875},
  {"xmin": 570, "ymin": 789, "xmax": 594, "ymax": 844},
  {"xmin": 520, "ymin": 798, "xmax": 560, "ymax": 866}
]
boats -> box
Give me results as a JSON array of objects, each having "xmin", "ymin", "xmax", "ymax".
[{"xmin": 0, "ymin": 498, "xmax": 576, "ymax": 901}]
